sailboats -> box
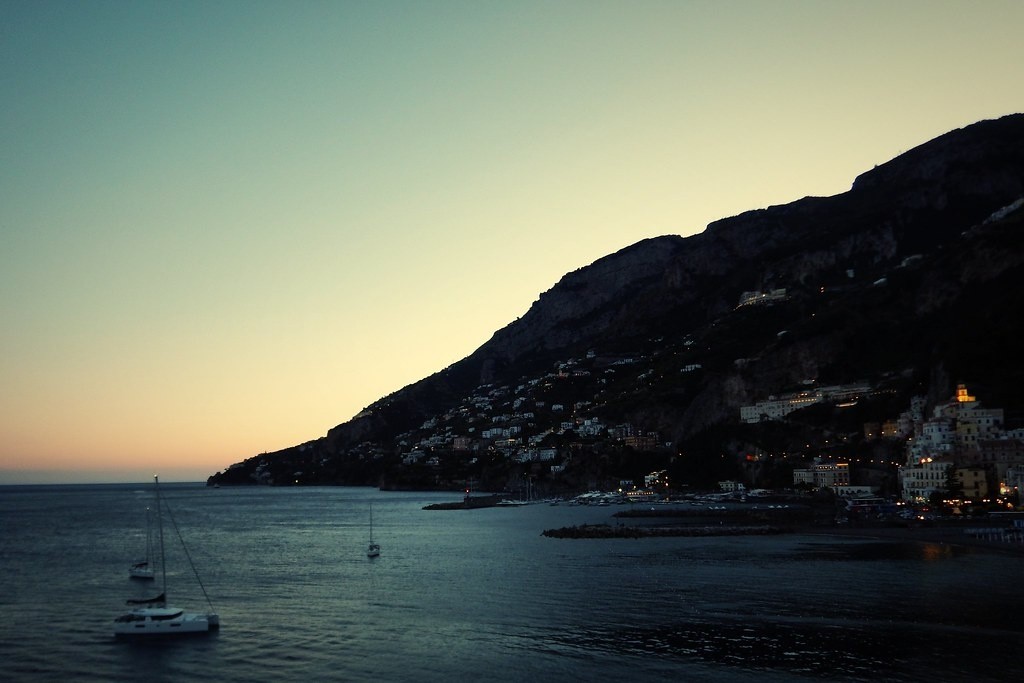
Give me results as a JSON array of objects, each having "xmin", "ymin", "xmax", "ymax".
[
  {"xmin": 110, "ymin": 475, "xmax": 221, "ymax": 635},
  {"xmin": 128, "ymin": 508, "xmax": 156, "ymax": 578},
  {"xmin": 367, "ymin": 503, "xmax": 382, "ymax": 558}
]
